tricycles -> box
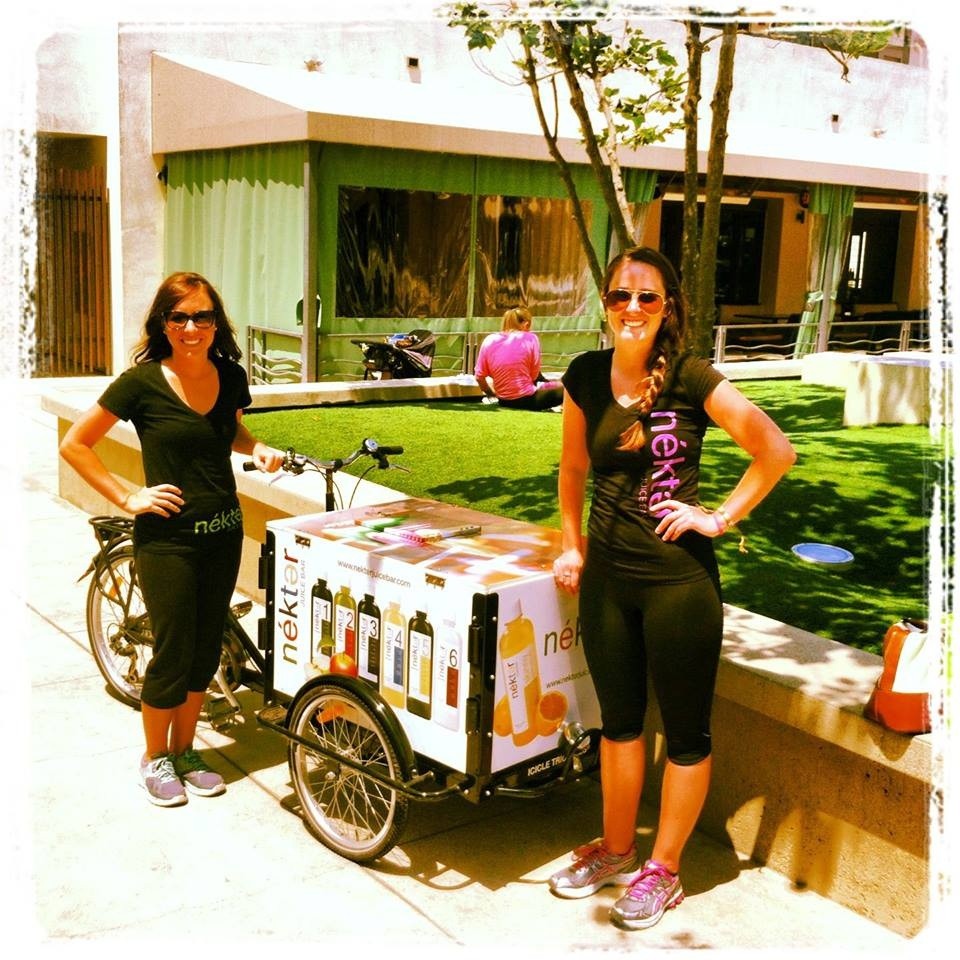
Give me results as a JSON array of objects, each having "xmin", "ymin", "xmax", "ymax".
[{"xmin": 77, "ymin": 438, "xmax": 620, "ymax": 866}]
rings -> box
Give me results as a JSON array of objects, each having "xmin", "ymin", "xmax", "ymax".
[{"xmin": 564, "ymin": 572, "xmax": 571, "ymax": 577}]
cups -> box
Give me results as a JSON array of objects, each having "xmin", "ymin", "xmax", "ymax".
[{"xmin": 534, "ymin": 690, "xmax": 569, "ymax": 737}]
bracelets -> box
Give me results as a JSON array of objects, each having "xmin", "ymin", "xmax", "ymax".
[
  {"xmin": 710, "ymin": 506, "xmax": 750, "ymax": 556},
  {"xmin": 118, "ymin": 492, "xmax": 134, "ymax": 507}
]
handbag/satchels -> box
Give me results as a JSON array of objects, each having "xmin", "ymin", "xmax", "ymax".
[{"xmin": 861, "ymin": 617, "xmax": 932, "ymax": 735}]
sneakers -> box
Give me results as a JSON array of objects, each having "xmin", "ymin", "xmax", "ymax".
[
  {"xmin": 548, "ymin": 843, "xmax": 642, "ymax": 898},
  {"xmin": 166, "ymin": 750, "xmax": 226, "ymax": 797},
  {"xmin": 137, "ymin": 752, "xmax": 188, "ymax": 806},
  {"xmin": 606, "ymin": 858, "xmax": 685, "ymax": 931}
]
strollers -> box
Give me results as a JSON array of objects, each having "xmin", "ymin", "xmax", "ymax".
[{"xmin": 349, "ymin": 329, "xmax": 437, "ymax": 381}]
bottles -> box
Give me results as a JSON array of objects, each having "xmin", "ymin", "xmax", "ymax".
[
  {"xmin": 353, "ymin": 593, "xmax": 379, "ymax": 688},
  {"xmin": 405, "ymin": 607, "xmax": 436, "ymax": 720},
  {"xmin": 435, "ymin": 621, "xmax": 462, "ymax": 730},
  {"xmin": 377, "ymin": 599, "xmax": 407, "ymax": 712},
  {"xmin": 501, "ymin": 598, "xmax": 542, "ymax": 747},
  {"xmin": 332, "ymin": 584, "xmax": 357, "ymax": 683},
  {"xmin": 307, "ymin": 575, "xmax": 335, "ymax": 676}
]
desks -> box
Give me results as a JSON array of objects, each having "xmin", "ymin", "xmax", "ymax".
[
  {"xmin": 734, "ymin": 314, "xmax": 794, "ymax": 333},
  {"xmin": 843, "ymin": 351, "xmax": 951, "ymax": 427}
]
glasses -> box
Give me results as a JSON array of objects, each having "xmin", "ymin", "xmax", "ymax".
[
  {"xmin": 161, "ymin": 311, "xmax": 215, "ymax": 329},
  {"xmin": 602, "ymin": 288, "xmax": 669, "ymax": 316}
]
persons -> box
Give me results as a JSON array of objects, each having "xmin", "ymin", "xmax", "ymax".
[
  {"xmin": 552, "ymin": 245, "xmax": 796, "ymax": 933},
  {"xmin": 61, "ymin": 272, "xmax": 284, "ymax": 808},
  {"xmin": 475, "ymin": 306, "xmax": 564, "ymax": 411}
]
